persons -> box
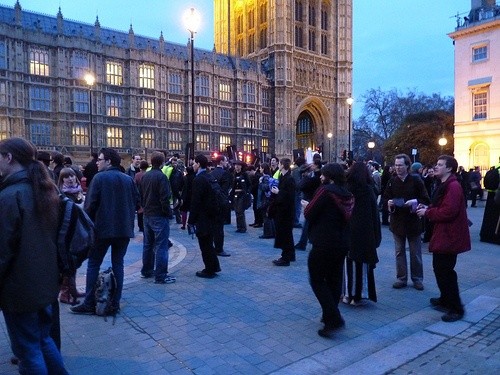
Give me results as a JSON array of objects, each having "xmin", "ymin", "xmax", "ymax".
[
  {"xmin": 13, "ymin": 149, "xmax": 500, "ymax": 367},
  {"xmin": 0, "ymin": 139, "xmax": 70, "ymax": 375}
]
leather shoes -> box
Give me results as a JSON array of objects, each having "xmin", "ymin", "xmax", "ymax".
[{"xmin": 196, "ymin": 269, "xmax": 221, "ymax": 278}]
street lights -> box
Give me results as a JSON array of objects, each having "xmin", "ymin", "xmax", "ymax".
[
  {"xmin": 327, "ymin": 131, "xmax": 333, "ymax": 163},
  {"xmin": 438, "ymin": 137, "xmax": 448, "ymax": 154},
  {"xmin": 84, "ymin": 73, "xmax": 96, "ymax": 159},
  {"xmin": 183, "ymin": 7, "xmax": 202, "ymax": 156},
  {"xmin": 346, "ymin": 94, "xmax": 354, "ymax": 158},
  {"xmin": 367, "ymin": 139, "xmax": 376, "ymax": 159},
  {"xmin": 248, "ymin": 115, "xmax": 254, "ymax": 153}
]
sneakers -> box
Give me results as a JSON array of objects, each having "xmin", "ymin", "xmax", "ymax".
[
  {"xmin": 141, "ymin": 270, "xmax": 156, "ymax": 277},
  {"xmin": 68, "ymin": 300, "xmax": 95, "ymax": 315},
  {"xmin": 153, "ymin": 274, "xmax": 176, "ymax": 284}
]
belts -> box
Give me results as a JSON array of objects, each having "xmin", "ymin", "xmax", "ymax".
[{"xmin": 235, "ymin": 189, "xmax": 243, "ymax": 192}]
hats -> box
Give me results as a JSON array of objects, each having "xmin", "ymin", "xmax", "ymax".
[{"xmin": 232, "ymin": 160, "xmax": 248, "ymax": 167}]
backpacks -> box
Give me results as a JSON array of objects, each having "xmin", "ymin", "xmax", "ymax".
[
  {"xmin": 197, "ymin": 173, "xmax": 231, "ymax": 224},
  {"xmin": 58, "ymin": 193, "xmax": 95, "ymax": 269},
  {"xmin": 92, "ymin": 267, "xmax": 117, "ymax": 317}
]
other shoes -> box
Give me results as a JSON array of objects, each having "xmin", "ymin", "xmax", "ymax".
[
  {"xmin": 382, "ymin": 221, "xmax": 390, "ymax": 225},
  {"xmin": 72, "ymin": 289, "xmax": 86, "ymax": 298},
  {"xmin": 342, "ymin": 296, "xmax": 370, "ymax": 306},
  {"xmin": 393, "ymin": 282, "xmax": 424, "ymax": 290},
  {"xmin": 235, "ymin": 229, "xmax": 246, "ymax": 233},
  {"xmin": 293, "ymin": 223, "xmax": 302, "ymax": 228},
  {"xmin": 181, "ymin": 227, "xmax": 185, "ymax": 229},
  {"xmin": 272, "ymin": 258, "xmax": 290, "ymax": 266},
  {"xmin": 259, "ymin": 235, "xmax": 266, "ymax": 238},
  {"xmin": 249, "ymin": 223, "xmax": 263, "ymax": 227},
  {"xmin": 294, "ymin": 243, "xmax": 306, "ymax": 250},
  {"xmin": 430, "ymin": 297, "xmax": 463, "ymax": 322},
  {"xmin": 177, "ymin": 221, "xmax": 182, "ymax": 224},
  {"xmin": 60, "ymin": 291, "xmax": 80, "ymax": 304},
  {"xmin": 318, "ymin": 317, "xmax": 345, "ymax": 336},
  {"xmin": 217, "ymin": 250, "xmax": 230, "ymax": 256}
]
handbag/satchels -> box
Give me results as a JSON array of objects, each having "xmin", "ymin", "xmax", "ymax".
[{"xmin": 238, "ymin": 194, "xmax": 251, "ymax": 209}]
